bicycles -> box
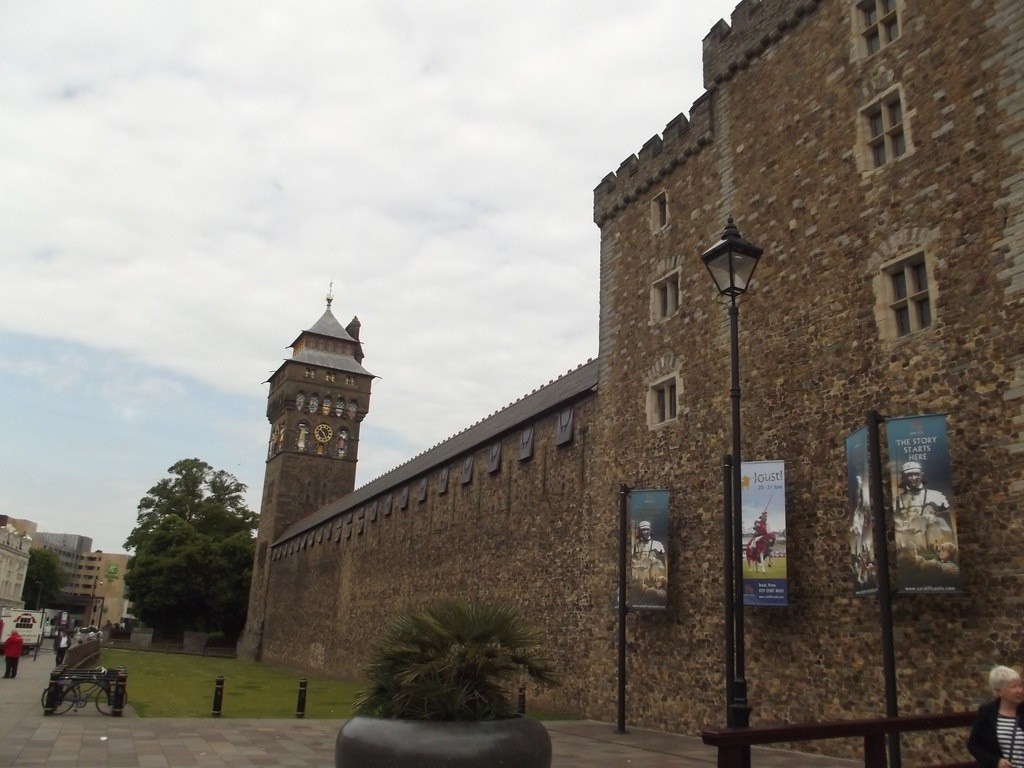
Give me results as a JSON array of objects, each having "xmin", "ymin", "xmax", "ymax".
[{"xmin": 41, "ymin": 664, "xmax": 128, "ymax": 716}]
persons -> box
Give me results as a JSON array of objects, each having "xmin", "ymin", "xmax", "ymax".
[
  {"xmin": 631, "ymin": 521, "xmax": 668, "ymax": 600},
  {"xmin": 967, "ymin": 665, "xmax": 1024, "ymax": 768},
  {"xmin": 55, "ymin": 628, "xmax": 71, "ymax": 667},
  {"xmin": 892, "ymin": 461, "xmax": 959, "ymax": 583},
  {"xmin": 2, "ymin": 629, "xmax": 24, "ymax": 678},
  {"xmin": 87, "ymin": 627, "xmax": 97, "ymax": 641}
]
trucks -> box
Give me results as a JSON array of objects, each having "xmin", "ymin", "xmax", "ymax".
[{"xmin": 0, "ymin": 608, "xmax": 46, "ymax": 655}]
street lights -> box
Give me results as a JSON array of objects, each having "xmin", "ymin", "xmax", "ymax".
[{"xmin": 701, "ymin": 213, "xmax": 763, "ymax": 767}]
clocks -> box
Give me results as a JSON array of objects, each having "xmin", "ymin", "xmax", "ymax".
[{"xmin": 314, "ymin": 423, "xmax": 333, "ymax": 443}]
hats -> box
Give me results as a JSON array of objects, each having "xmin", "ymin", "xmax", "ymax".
[
  {"xmin": 637, "ymin": 520, "xmax": 651, "ymax": 529},
  {"xmin": 925, "ymin": 501, "xmax": 940, "ymax": 514},
  {"xmin": 760, "ymin": 512, "xmax": 768, "ymax": 519},
  {"xmin": 902, "ymin": 462, "xmax": 923, "ymax": 490},
  {"xmin": 657, "ymin": 576, "xmax": 667, "ymax": 587},
  {"xmin": 903, "ymin": 541, "xmax": 918, "ymax": 550}
]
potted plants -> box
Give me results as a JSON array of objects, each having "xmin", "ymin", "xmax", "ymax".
[{"xmin": 334, "ymin": 594, "xmax": 564, "ymax": 768}]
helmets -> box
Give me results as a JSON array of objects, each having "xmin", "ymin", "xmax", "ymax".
[{"xmin": 940, "ymin": 541, "xmax": 957, "ymax": 556}]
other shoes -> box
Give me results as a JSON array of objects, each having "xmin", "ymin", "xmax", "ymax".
[
  {"xmin": 2, "ymin": 675, "xmax": 10, "ymax": 678},
  {"xmin": 749, "ymin": 547, "xmax": 756, "ymax": 555},
  {"xmin": 11, "ymin": 674, "xmax": 16, "ymax": 678}
]
